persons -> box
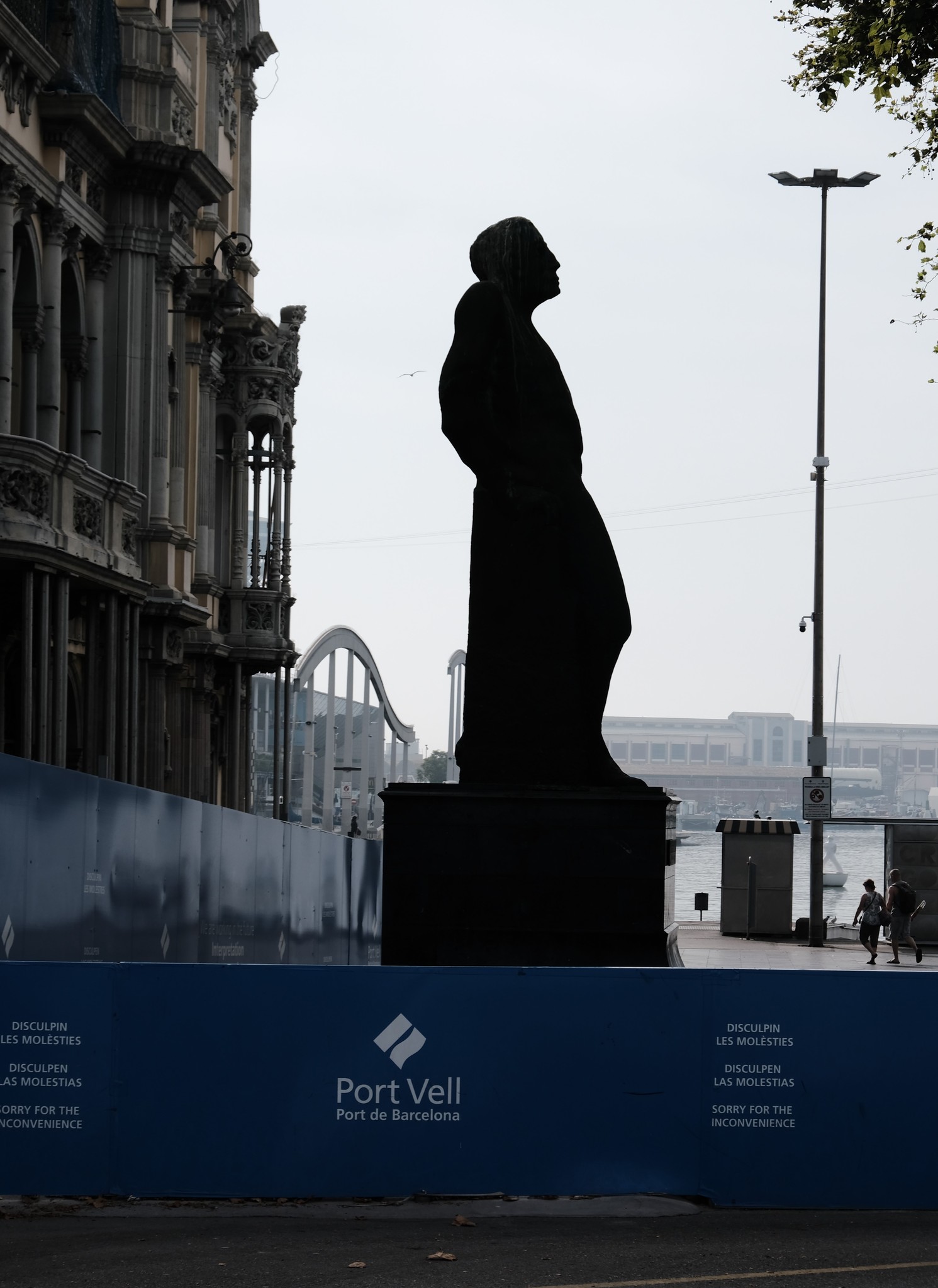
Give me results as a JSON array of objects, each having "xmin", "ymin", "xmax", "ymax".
[
  {"xmin": 886, "ymin": 868, "xmax": 922, "ymax": 964},
  {"xmin": 853, "ymin": 880, "xmax": 893, "ymax": 964},
  {"xmin": 442, "ymin": 217, "xmax": 645, "ymax": 787}
]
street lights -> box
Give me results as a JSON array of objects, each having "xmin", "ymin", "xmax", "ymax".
[{"xmin": 767, "ymin": 166, "xmax": 883, "ymax": 946}]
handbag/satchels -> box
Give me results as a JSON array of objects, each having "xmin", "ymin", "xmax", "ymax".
[
  {"xmin": 858, "ymin": 916, "xmax": 862, "ymax": 924},
  {"xmin": 878, "ymin": 908, "xmax": 891, "ymax": 927}
]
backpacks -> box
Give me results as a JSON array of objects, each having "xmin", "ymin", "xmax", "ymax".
[{"xmin": 891, "ymin": 883, "xmax": 915, "ymax": 914}]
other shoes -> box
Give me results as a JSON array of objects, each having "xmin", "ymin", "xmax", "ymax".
[
  {"xmin": 867, "ymin": 961, "xmax": 876, "ymax": 965},
  {"xmin": 871, "ymin": 953, "xmax": 878, "ymax": 960}
]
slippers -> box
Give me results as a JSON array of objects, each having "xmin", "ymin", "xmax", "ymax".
[
  {"xmin": 915, "ymin": 949, "xmax": 922, "ymax": 963},
  {"xmin": 886, "ymin": 959, "xmax": 900, "ymax": 964}
]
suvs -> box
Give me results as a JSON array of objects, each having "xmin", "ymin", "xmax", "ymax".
[{"xmin": 845, "ymin": 807, "xmax": 867, "ymax": 816}]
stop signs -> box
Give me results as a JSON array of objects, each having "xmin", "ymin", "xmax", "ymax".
[{"xmin": 352, "ymin": 799, "xmax": 357, "ymax": 805}]
